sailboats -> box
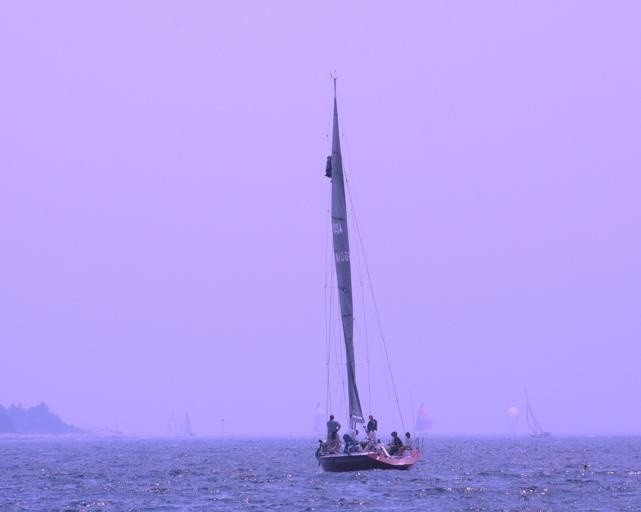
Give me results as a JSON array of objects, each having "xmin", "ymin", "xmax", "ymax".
[
  {"xmin": 524, "ymin": 388, "xmax": 553, "ymax": 439},
  {"xmin": 317, "ymin": 71, "xmax": 424, "ymax": 472}
]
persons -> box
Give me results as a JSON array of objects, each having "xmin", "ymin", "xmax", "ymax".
[
  {"xmin": 367, "ymin": 415, "xmax": 377, "ymax": 445},
  {"xmin": 343, "ymin": 429, "xmax": 359, "ymax": 454},
  {"xmin": 405, "ymin": 432, "xmax": 412, "ymax": 450},
  {"xmin": 388, "ymin": 432, "xmax": 403, "ymax": 456},
  {"xmin": 326, "ymin": 415, "xmax": 341, "ymax": 441}
]
